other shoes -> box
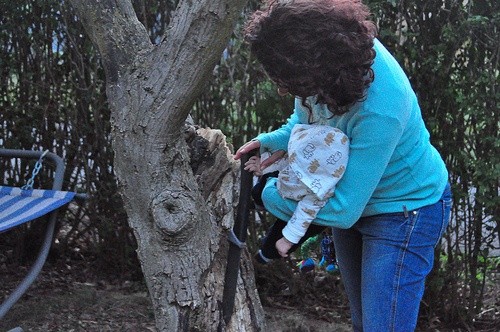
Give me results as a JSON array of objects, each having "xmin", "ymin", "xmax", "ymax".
[{"xmin": 255, "ymin": 248, "xmax": 270, "ymax": 263}]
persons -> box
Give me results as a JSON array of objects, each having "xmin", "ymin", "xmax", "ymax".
[
  {"xmin": 235, "ymin": 0, "xmax": 455, "ymax": 332},
  {"xmin": 244, "ymin": 122, "xmax": 351, "ymax": 263}
]
